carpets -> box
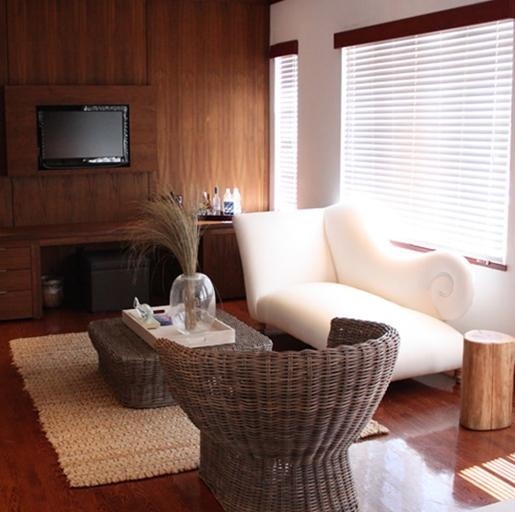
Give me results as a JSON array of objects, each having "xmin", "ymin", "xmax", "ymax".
[{"xmin": 8, "ymin": 324, "xmax": 388, "ymax": 490}]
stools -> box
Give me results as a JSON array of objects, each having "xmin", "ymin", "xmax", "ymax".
[
  {"xmin": 459, "ymin": 329, "xmax": 514, "ymax": 432},
  {"xmin": 68, "ymin": 250, "xmax": 151, "ymax": 314}
]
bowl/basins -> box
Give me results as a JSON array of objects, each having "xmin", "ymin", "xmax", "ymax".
[{"xmin": 39, "ymin": 271, "xmax": 76, "ymax": 308}]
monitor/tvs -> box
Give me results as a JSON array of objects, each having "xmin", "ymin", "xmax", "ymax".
[{"xmin": 36, "ymin": 104, "xmax": 130, "ymax": 169}]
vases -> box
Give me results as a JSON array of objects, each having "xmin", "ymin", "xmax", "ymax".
[{"xmin": 170, "ymin": 271, "xmax": 216, "ymax": 337}]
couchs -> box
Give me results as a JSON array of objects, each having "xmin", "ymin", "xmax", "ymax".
[{"xmin": 233, "ymin": 200, "xmax": 474, "ymax": 383}]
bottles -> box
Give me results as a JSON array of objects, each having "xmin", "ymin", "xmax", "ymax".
[{"xmin": 199, "ymin": 185, "xmax": 241, "ymax": 218}]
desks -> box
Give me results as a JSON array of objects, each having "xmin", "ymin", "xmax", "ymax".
[
  {"xmin": 87, "ymin": 307, "xmax": 273, "ymax": 408},
  {"xmin": 0, "ymin": 219, "xmax": 245, "ymax": 323}
]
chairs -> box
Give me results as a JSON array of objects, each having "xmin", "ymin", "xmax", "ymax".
[{"xmin": 155, "ymin": 317, "xmax": 399, "ymax": 512}]
